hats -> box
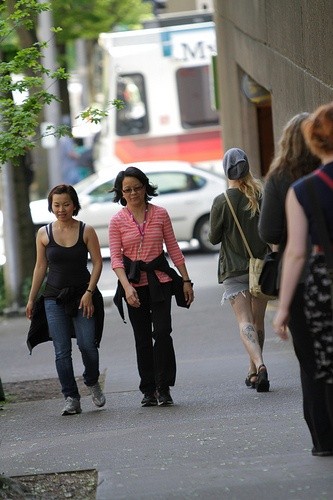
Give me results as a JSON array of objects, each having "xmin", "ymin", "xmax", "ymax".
[{"xmin": 223, "ymin": 147, "xmax": 250, "ymax": 179}]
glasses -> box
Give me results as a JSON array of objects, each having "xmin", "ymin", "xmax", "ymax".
[{"xmin": 122, "ymin": 184, "xmax": 145, "ymax": 194}]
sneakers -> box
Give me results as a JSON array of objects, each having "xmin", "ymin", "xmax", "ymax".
[
  {"xmin": 89, "ymin": 382, "xmax": 105, "ymax": 407},
  {"xmin": 141, "ymin": 391, "xmax": 158, "ymax": 408},
  {"xmin": 62, "ymin": 397, "xmax": 82, "ymax": 415},
  {"xmin": 156, "ymin": 391, "xmax": 173, "ymax": 407}
]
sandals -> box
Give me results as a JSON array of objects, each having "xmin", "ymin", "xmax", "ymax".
[
  {"xmin": 256, "ymin": 365, "xmax": 269, "ymax": 392},
  {"xmin": 245, "ymin": 371, "xmax": 257, "ymax": 388}
]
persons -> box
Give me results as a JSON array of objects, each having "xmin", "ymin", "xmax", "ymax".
[
  {"xmin": 209, "ymin": 148, "xmax": 275, "ymax": 393},
  {"xmin": 108, "ymin": 168, "xmax": 194, "ymax": 408},
  {"xmin": 58, "ymin": 125, "xmax": 95, "ymax": 185},
  {"xmin": 273, "ymin": 103, "xmax": 333, "ymax": 386},
  {"xmin": 26, "ymin": 185, "xmax": 106, "ymax": 416},
  {"xmin": 258, "ymin": 112, "xmax": 333, "ymax": 456}
]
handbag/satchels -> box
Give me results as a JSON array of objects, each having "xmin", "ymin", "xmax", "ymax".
[{"xmin": 249, "ymin": 257, "xmax": 280, "ymax": 300}]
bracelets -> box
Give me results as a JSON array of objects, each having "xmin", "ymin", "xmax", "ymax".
[
  {"xmin": 87, "ymin": 289, "xmax": 94, "ymax": 294},
  {"xmin": 183, "ymin": 280, "xmax": 191, "ymax": 283}
]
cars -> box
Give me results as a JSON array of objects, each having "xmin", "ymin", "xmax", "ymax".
[{"xmin": 28, "ymin": 161, "xmax": 229, "ymax": 253}]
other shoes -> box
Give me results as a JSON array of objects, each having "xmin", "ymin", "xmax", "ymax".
[{"xmin": 312, "ymin": 446, "xmax": 331, "ymax": 457}]
